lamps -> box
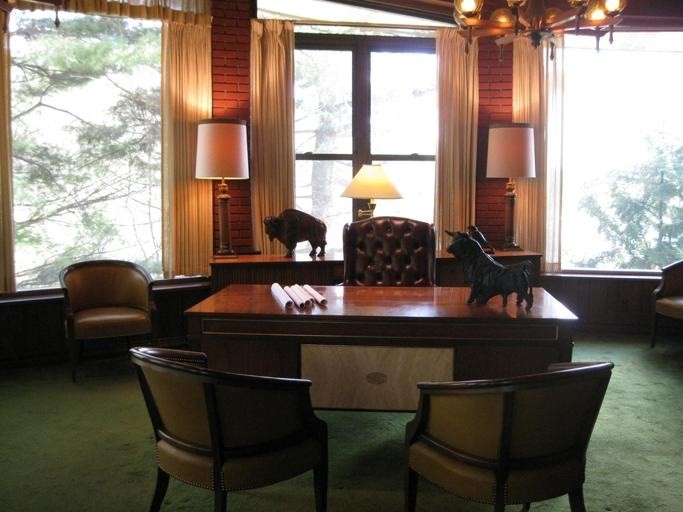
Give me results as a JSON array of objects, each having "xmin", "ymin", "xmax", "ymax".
[
  {"xmin": 194, "ymin": 120, "xmax": 249, "ymax": 258},
  {"xmin": 453, "ymin": 0, "xmax": 627, "ymax": 62},
  {"xmin": 486, "ymin": 122, "xmax": 536, "ymax": 252},
  {"xmin": 341, "ymin": 164, "xmax": 402, "ymax": 219}
]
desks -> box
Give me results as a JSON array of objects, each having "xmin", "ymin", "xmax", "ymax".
[
  {"xmin": 185, "ymin": 283, "xmax": 578, "ymax": 412},
  {"xmin": 208, "ymin": 250, "xmax": 543, "ymax": 295}
]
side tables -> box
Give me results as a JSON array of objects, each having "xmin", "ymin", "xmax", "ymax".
[{"xmin": 152, "ymin": 281, "xmax": 209, "ymax": 345}]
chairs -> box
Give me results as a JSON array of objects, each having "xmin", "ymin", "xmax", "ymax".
[
  {"xmin": 650, "ymin": 261, "xmax": 683, "ymax": 348},
  {"xmin": 342, "ymin": 216, "xmax": 436, "ymax": 288},
  {"xmin": 404, "ymin": 361, "xmax": 614, "ymax": 512},
  {"xmin": 59, "ymin": 261, "xmax": 155, "ymax": 383},
  {"xmin": 130, "ymin": 347, "xmax": 328, "ymax": 512}
]
